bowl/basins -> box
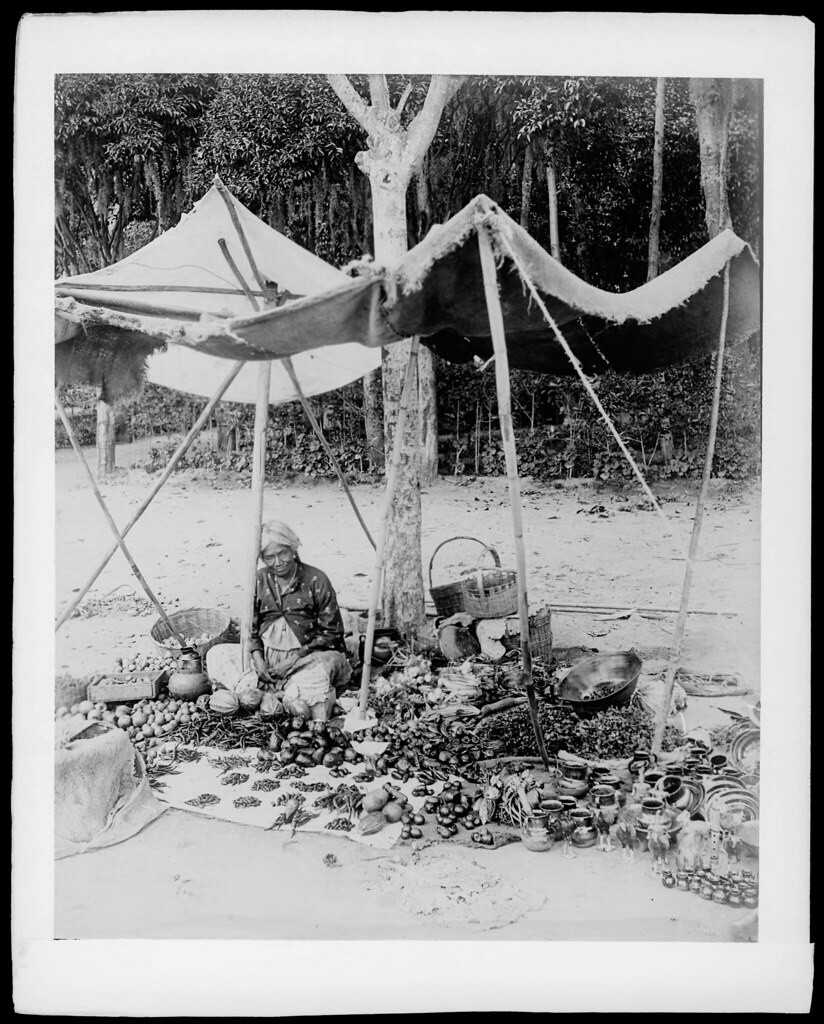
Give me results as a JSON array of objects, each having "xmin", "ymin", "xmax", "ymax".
[{"xmin": 636, "ymin": 699, "xmax": 760, "ymax": 853}]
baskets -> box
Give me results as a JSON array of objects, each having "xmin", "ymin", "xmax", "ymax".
[
  {"xmin": 427, "ymin": 536, "xmax": 501, "ymax": 619},
  {"xmin": 461, "ymin": 546, "xmax": 518, "ymax": 620},
  {"xmin": 475, "ymin": 600, "xmax": 554, "ymax": 668},
  {"xmin": 358, "ymin": 610, "xmax": 383, "ymax": 634},
  {"xmin": 148, "ymin": 607, "xmax": 232, "ymax": 666}
]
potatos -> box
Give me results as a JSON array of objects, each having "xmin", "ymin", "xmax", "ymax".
[{"xmin": 55, "ymin": 632, "xmax": 211, "ymax": 767}]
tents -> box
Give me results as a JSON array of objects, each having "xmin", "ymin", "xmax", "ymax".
[{"xmin": 56, "ymin": 172, "xmax": 765, "ymax": 780}]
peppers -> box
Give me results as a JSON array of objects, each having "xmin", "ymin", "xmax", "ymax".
[{"xmin": 161, "ymin": 712, "xmax": 281, "ymax": 770}]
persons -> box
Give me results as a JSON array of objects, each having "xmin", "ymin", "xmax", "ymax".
[{"xmin": 205, "ymin": 520, "xmax": 353, "ymax": 710}]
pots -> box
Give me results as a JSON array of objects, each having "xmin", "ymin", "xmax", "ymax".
[{"xmin": 557, "ymin": 645, "xmax": 644, "ymax": 717}]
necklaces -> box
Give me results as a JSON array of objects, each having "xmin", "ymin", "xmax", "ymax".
[{"xmin": 273, "ymin": 562, "xmax": 298, "ymax": 605}]
